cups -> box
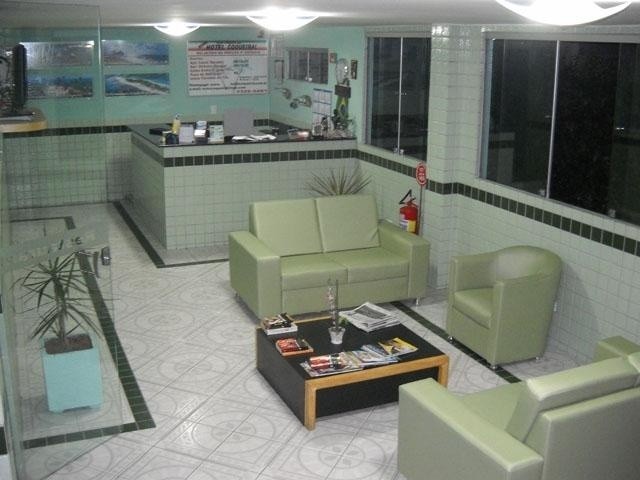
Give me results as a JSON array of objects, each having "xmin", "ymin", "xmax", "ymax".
[
  {"xmin": 272, "ymin": 127, "xmax": 280, "ymax": 136},
  {"xmin": 287, "ymin": 129, "xmax": 299, "ymax": 140}
]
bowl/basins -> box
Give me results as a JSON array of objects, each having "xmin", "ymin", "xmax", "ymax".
[{"xmin": 298, "ymin": 130, "xmax": 310, "ymax": 138}]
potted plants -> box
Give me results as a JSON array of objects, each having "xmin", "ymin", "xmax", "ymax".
[{"xmin": 9, "ymin": 225, "xmax": 104, "ymax": 412}]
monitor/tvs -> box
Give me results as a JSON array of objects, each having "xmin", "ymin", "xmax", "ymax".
[{"xmin": 12, "ymin": 44, "xmax": 28, "ymax": 116}]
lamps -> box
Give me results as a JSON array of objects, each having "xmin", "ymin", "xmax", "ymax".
[
  {"xmin": 249, "ymin": 12, "xmax": 317, "ymax": 30},
  {"xmin": 154, "ymin": 22, "xmax": 199, "ymax": 37},
  {"xmin": 497, "ymin": 0, "xmax": 635, "ymax": 26}
]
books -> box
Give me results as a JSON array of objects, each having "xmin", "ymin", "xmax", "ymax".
[
  {"xmin": 299, "ymin": 351, "xmax": 398, "ymax": 378},
  {"xmin": 362, "ymin": 338, "xmax": 418, "ymax": 359},
  {"xmin": 276, "ymin": 338, "xmax": 313, "ymax": 356},
  {"xmin": 260, "ymin": 312, "xmax": 298, "ymax": 335},
  {"xmin": 208, "ymin": 125, "xmax": 224, "ymax": 144}
]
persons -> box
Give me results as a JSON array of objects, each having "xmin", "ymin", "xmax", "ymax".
[{"xmin": 378, "ymin": 337, "xmax": 419, "ymax": 356}]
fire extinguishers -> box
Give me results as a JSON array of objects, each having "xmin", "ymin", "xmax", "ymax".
[{"xmin": 400, "ymin": 198, "xmax": 418, "ymax": 234}]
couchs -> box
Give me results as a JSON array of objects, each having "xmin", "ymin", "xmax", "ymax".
[
  {"xmin": 449, "ymin": 245, "xmax": 562, "ymax": 370},
  {"xmin": 396, "ymin": 336, "xmax": 640, "ymax": 480},
  {"xmin": 229, "ymin": 194, "xmax": 429, "ymax": 320}
]
